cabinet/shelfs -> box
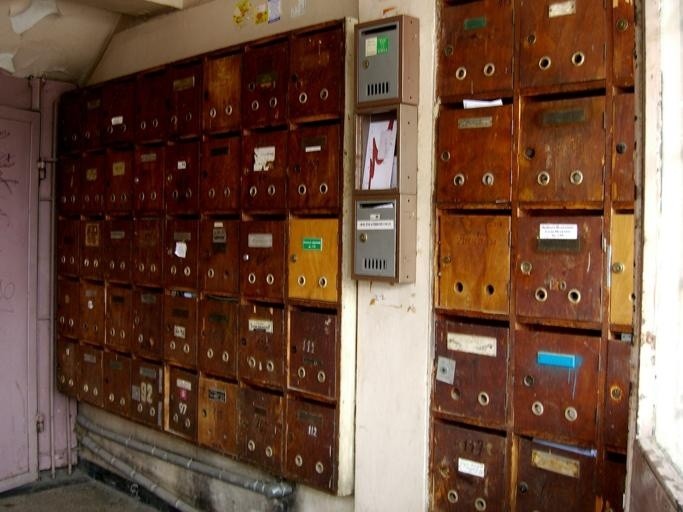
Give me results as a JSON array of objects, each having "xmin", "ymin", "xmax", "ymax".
[
  {"xmin": 55, "ymin": 14, "xmax": 355, "ymax": 497},
  {"xmin": 430, "ymin": 1, "xmax": 640, "ymax": 508}
]
